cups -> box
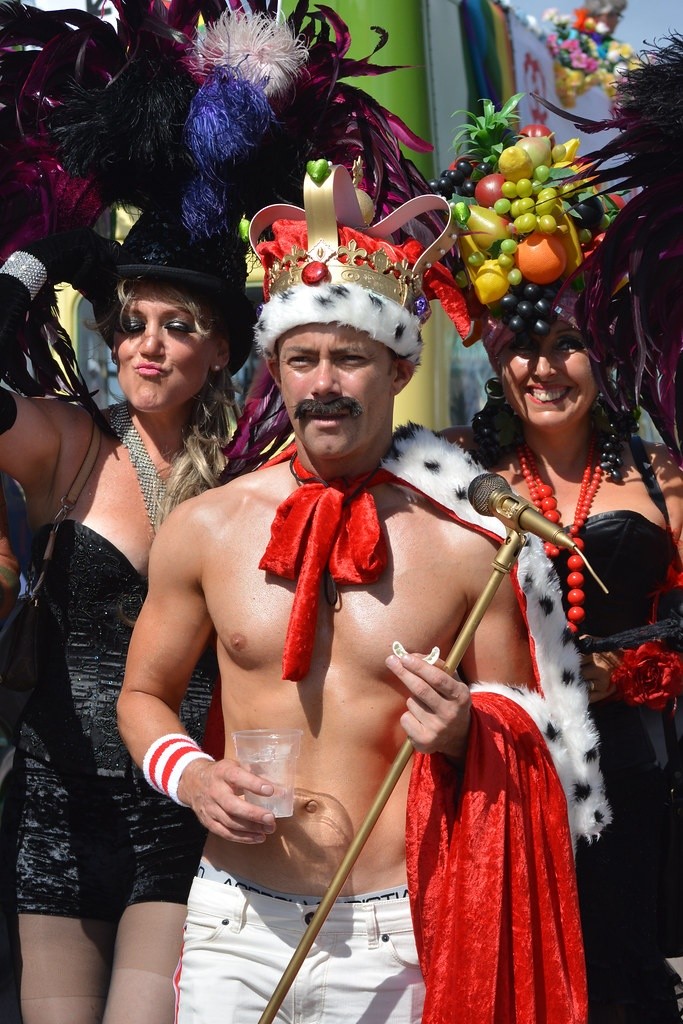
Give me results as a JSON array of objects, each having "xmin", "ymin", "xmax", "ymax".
[{"xmin": 232, "ymin": 727, "xmax": 303, "ymax": 818}]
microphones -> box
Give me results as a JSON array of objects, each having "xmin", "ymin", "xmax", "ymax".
[{"xmin": 468, "ymin": 473, "xmax": 609, "ymax": 601}]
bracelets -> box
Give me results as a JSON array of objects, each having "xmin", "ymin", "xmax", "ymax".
[{"xmin": 146, "ymin": 734, "xmax": 214, "ymax": 807}]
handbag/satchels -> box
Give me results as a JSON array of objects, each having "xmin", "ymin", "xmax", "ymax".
[{"xmin": 0, "ymin": 407, "xmax": 101, "ymax": 744}]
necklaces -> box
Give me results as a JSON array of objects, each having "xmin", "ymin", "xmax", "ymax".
[
  {"xmin": 119, "ymin": 419, "xmax": 168, "ymax": 532},
  {"xmin": 527, "ymin": 448, "xmax": 602, "ymax": 557},
  {"xmin": 516, "ymin": 447, "xmax": 602, "ymax": 632}
]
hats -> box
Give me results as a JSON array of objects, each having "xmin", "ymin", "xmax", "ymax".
[{"xmin": 240, "ymin": 159, "xmax": 456, "ymax": 365}]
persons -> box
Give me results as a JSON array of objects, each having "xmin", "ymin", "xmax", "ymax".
[
  {"xmin": 114, "ymin": 158, "xmax": 548, "ymax": 1023},
  {"xmin": 0, "ymin": 0, "xmax": 683, "ymax": 1024}
]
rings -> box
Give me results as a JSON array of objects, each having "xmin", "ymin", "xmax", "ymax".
[{"xmin": 589, "ymin": 679, "xmax": 594, "ymax": 692}]
